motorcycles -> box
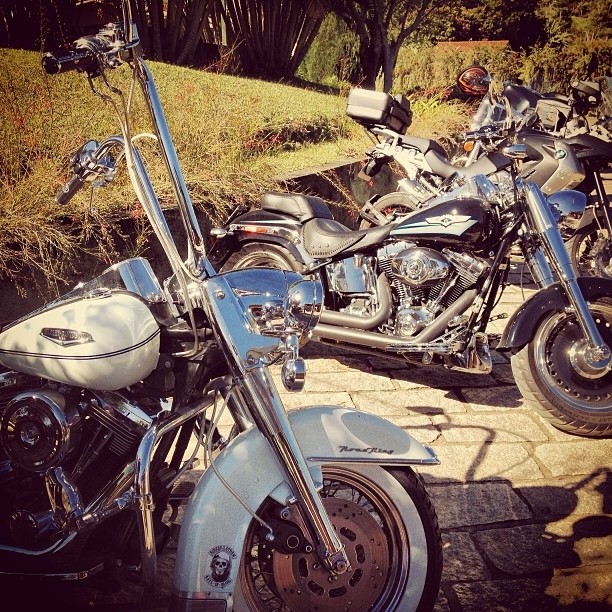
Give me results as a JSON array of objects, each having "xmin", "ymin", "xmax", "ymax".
[
  {"xmin": 439, "ymin": 64, "xmax": 600, "ymax": 196},
  {"xmin": 344, "ymin": 79, "xmax": 611, "ymax": 283},
  {"xmin": 2, "ymin": 1, "xmax": 448, "ymax": 611},
  {"xmin": 198, "ymin": 96, "xmax": 612, "ymax": 437}
]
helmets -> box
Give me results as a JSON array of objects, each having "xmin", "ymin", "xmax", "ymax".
[{"xmin": 456, "ymin": 65, "xmax": 490, "ymax": 99}]
